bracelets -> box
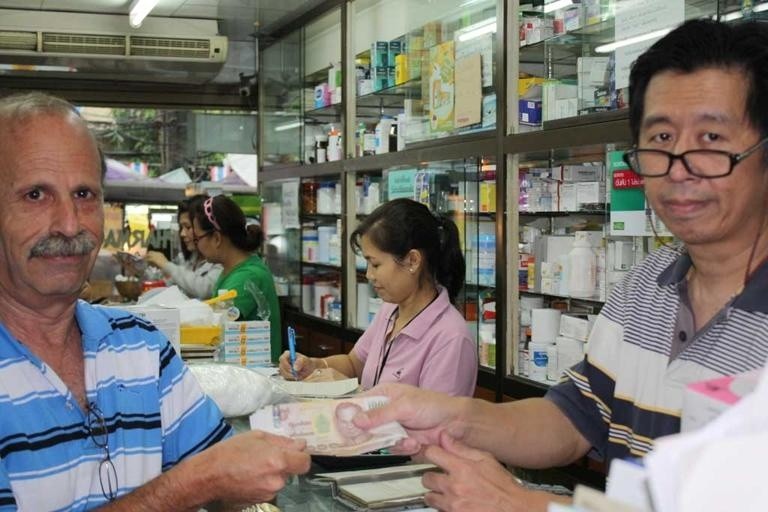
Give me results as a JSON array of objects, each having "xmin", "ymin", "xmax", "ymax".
[{"xmin": 323, "ymin": 358, "xmax": 329, "ymax": 368}]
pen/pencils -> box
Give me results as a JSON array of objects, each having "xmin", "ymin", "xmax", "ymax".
[{"xmin": 288, "ymin": 326, "xmax": 297, "ymax": 381}]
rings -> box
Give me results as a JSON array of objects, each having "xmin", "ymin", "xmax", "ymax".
[{"xmin": 315, "ymin": 370, "xmax": 321, "ymax": 376}]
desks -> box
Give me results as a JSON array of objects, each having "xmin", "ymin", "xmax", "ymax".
[{"xmin": 177, "ymin": 349, "xmax": 574, "ymax": 511}]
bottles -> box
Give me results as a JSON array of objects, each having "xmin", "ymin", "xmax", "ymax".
[
  {"xmin": 566, "ymin": 239, "xmax": 598, "ymax": 300},
  {"xmin": 523, "ymin": 329, "xmax": 532, "ymax": 378},
  {"xmin": 313, "ymin": 134, "xmax": 328, "ymax": 164},
  {"xmin": 481, "ymin": 163, "xmax": 496, "ymax": 185},
  {"xmin": 362, "ymin": 116, "xmax": 397, "ymax": 157}
]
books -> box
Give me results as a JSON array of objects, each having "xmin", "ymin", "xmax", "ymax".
[{"xmin": 310, "ymin": 459, "xmax": 434, "ymax": 512}]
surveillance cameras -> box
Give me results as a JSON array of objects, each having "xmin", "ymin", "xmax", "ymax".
[{"xmin": 238, "ymin": 78, "xmax": 251, "ymax": 99}]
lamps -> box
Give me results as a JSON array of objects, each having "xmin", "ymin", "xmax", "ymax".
[{"xmin": 127, "ymin": 1, "xmax": 161, "ymax": 29}]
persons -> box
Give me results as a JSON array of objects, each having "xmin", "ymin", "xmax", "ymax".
[
  {"xmin": 190, "ymin": 194, "xmax": 283, "ymax": 364},
  {"xmin": 276, "ymin": 199, "xmax": 482, "ymax": 396},
  {"xmin": 353, "ymin": 14, "xmax": 767, "ymax": 472},
  {"xmin": 418, "ymin": 362, "xmax": 768, "ymax": 509},
  {"xmin": 144, "ymin": 194, "xmax": 222, "ymax": 299},
  {"xmin": 0, "ymin": 90, "xmax": 310, "ymax": 512}
]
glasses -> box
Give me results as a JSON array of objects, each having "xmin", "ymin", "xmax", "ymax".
[
  {"xmin": 621, "ymin": 134, "xmax": 768, "ymax": 180},
  {"xmin": 191, "ymin": 228, "xmax": 213, "ymax": 244},
  {"xmin": 85, "ymin": 401, "xmax": 120, "ymax": 502}
]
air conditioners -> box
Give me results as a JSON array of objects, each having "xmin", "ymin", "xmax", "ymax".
[{"xmin": 0, "ymin": 7, "xmax": 229, "ymax": 64}]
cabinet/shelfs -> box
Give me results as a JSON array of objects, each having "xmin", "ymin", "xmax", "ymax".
[
  {"xmin": 0, "ymin": 74, "xmax": 260, "ymax": 282},
  {"xmin": 257, "ymin": 0, "xmax": 633, "ymax": 496}
]
